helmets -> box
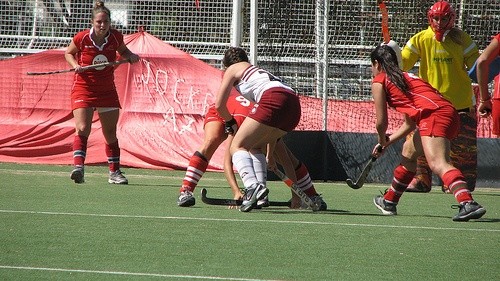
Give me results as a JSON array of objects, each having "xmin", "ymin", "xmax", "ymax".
[{"xmin": 428, "ymin": 1, "xmax": 456, "ymax": 42}]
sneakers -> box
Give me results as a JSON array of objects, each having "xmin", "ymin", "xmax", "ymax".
[
  {"xmin": 178, "ymin": 191, "xmax": 196, "ymax": 207},
  {"xmin": 70, "ymin": 165, "xmax": 85, "ymax": 184},
  {"xmin": 450, "ymin": 201, "xmax": 486, "ymax": 222},
  {"xmin": 108, "ymin": 170, "xmax": 128, "ymax": 184},
  {"xmin": 373, "ymin": 189, "xmax": 397, "ymax": 216},
  {"xmin": 240, "ymin": 182, "xmax": 270, "ymax": 212}
]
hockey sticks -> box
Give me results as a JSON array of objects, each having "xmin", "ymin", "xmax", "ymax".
[
  {"xmin": 28, "ymin": 57, "xmax": 137, "ymax": 76},
  {"xmin": 201, "ymin": 188, "xmax": 291, "ymax": 206},
  {"xmin": 376, "ymin": 0, "xmax": 390, "ymax": 44},
  {"xmin": 347, "ymin": 136, "xmax": 389, "ymax": 190},
  {"xmin": 225, "ymin": 127, "xmax": 322, "ymax": 212}
]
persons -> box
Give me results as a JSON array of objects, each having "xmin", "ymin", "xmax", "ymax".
[
  {"xmin": 394, "ymin": 0, "xmax": 496, "ymax": 195},
  {"xmin": 367, "ymin": 44, "xmax": 488, "ymax": 223},
  {"xmin": 64, "ymin": 1, "xmax": 141, "ymax": 186},
  {"xmin": 0, "ymin": 1, "xmax": 500, "ymax": 58},
  {"xmin": 175, "ymin": 42, "xmax": 330, "ymax": 215}
]
